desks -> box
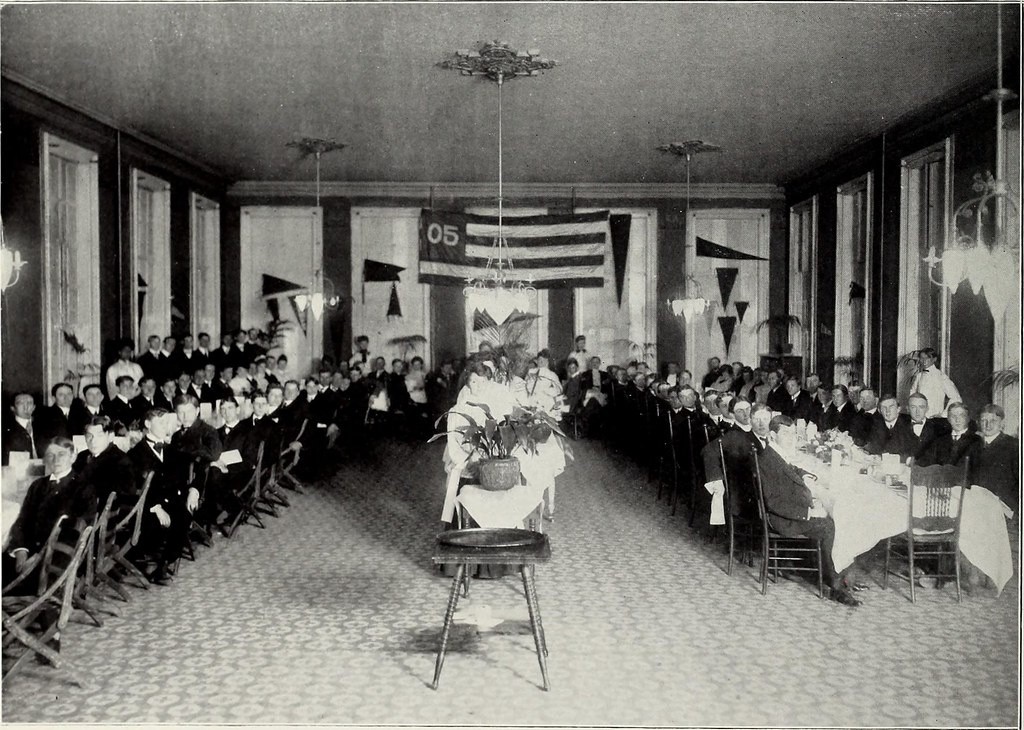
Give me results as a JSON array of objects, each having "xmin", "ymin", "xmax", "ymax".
[
  {"xmin": 792, "ymin": 442, "xmax": 1014, "ymax": 600},
  {"xmin": 2, "ymin": 457, "xmax": 42, "ymax": 552}
]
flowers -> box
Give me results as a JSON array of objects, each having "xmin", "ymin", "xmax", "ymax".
[{"xmin": 811, "ymin": 425, "xmax": 857, "ymax": 463}]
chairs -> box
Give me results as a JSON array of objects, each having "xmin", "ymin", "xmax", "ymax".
[
  {"xmin": 653, "ymin": 404, "xmax": 971, "ymax": 607},
  {"xmin": 1, "ymin": 418, "xmax": 310, "ymax": 693}
]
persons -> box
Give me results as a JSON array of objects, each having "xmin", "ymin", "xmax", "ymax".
[
  {"xmin": 0, "ymin": 328, "xmax": 1021, "ymax": 664},
  {"xmin": 909, "ymin": 348, "xmax": 962, "ymax": 419},
  {"xmin": 754, "ymin": 415, "xmax": 864, "ymax": 606},
  {"xmin": 349, "ymin": 336, "xmax": 375, "ymax": 377},
  {"xmin": 566, "ymin": 335, "xmax": 592, "ymax": 381}
]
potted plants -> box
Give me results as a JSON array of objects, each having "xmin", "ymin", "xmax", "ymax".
[
  {"xmin": 427, "ymin": 402, "xmax": 566, "ymax": 490},
  {"xmin": 756, "ymin": 311, "xmax": 802, "ymax": 352}
]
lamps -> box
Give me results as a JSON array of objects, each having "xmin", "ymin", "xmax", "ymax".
[
  {"xmin": 291, "ymin": 137, "xmax": 344, "ymax": 318},
  {"xmin": 923, "ymin": 4, "xmax": 1022, "ymax": 322},
  {"xmin": 657, "ymin": 139, "xmax": 718, "ymax": 318},
  {"xmin": 434, "ymin": 39, "xmax": 562, "ymax": 330}
]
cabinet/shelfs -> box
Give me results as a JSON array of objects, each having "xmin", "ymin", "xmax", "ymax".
[{"xmin": 760, "ymin": 355, "xmax": 803, "ymax": 382}]
